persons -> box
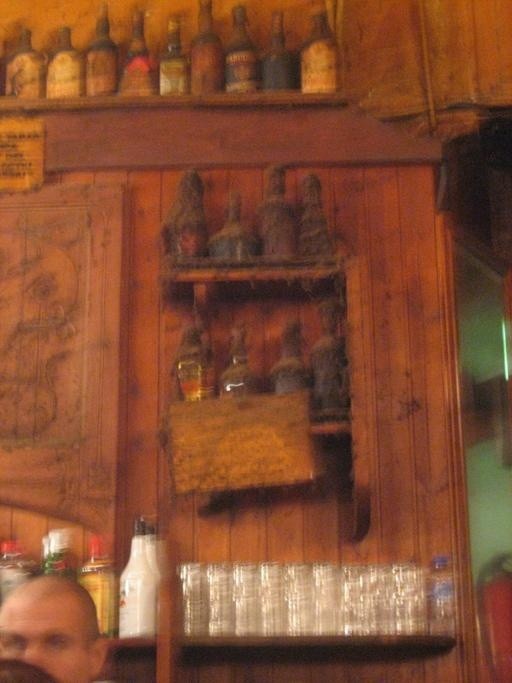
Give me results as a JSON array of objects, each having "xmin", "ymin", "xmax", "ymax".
[{"xmin": 0, "ymin": 574, "xmax": 109, "ymax": 683}]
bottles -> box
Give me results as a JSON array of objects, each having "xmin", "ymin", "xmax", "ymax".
[
  {"xmin": 299, "ymin": 174, "xmax": 332, "ymax": 254},
  {"xmin": 430, "ymin": 556, "xmax": 457, "ymax": 637},
  {"xmin": 188, "ymin": 1, "xmax": 221, "ymax": 94},
  {"xmin": 222, "ymin": 5, "xmax": 259, "ymax": 90},
  {"xmin": 9, "ymin": 30, "xmax": 44, "ymax": 97},
  {"xmin": 297, "ymin": 4, "xmax": 341, "ymax": 95},
  {"xmin": 222, "ymin": 326, "xmax": 261, "ymax": 394},
  {"xmin": 170, "ymin": 171, "xmax": 206, "ymax": 256},
  {"xmin": 42, "ymin": 529, "xmax": 75, "ymax": 580},
  {"xmin": 171, "ymin": 325, "xmax": 216, "ymax": 400},
  {"xmin": 158, "ymin": 15, "xmax": 187, "ymax": 96},
  {"xmin": 262, "ymin": 163, "xmax": 298, "ymax": 254},
  {"xmin": 45, "ymin": 27, "xmax": 82, "ymax": 97},
  {"xmin": 84, "ymin": 3, "xmax": 119, "ymax": 95},
  {"xmin": 215, "ymin": 191, "xmax": 259, "ymax": 256},
  {"xmin": 80, "ymin": 533, "xmax": 117, "ymax": 638},
  {"xmin": 119, "ymin": 12, "xmax": 152, "ymax": 94},
  {"xmin": 117, "ymin": 517, "xmax": 160, "ymax": 637},
  {"xmin": 316, "ymin": 300, "xmax": 352, "ymax": 416},
  {"xmin": 272, "ymin": 320, "xmax": 308, "ymax": 395},
  {"xmin": 260, "ymin": 9, "xmax": 296, "ymax": 88},
  {"xmin": 1, "ymin": 538, "xmax": 36, "ymax": 601}
]
